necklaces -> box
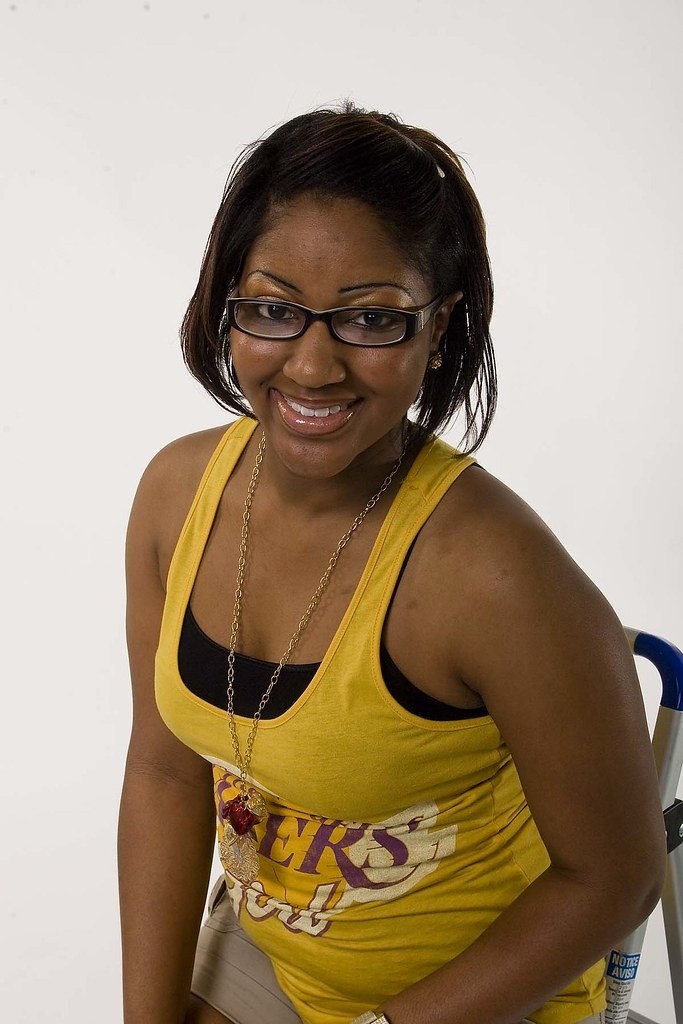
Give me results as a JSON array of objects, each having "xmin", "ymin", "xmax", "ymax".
[{"xmin": 217, "ymin": 433, "xmax": 410, "ymax": 886}]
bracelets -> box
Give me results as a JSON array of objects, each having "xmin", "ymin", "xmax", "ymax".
[{"xmin": 354, "ymin": 1010, "xmax": 389, "ymax": 1024}]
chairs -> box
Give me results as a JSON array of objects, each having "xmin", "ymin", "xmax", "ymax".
[{"xmin": 604, "ymin": 626, "xmax": 683, "ymax": 1024}]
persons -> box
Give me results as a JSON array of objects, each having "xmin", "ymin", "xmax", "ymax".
[{"xmin": 116, "ymin": 103, "xmax": 668, "ymax": 1024}]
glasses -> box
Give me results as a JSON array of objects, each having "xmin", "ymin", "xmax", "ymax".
[{"xmin": 225, "ymin": 279, "xmax": 443, "ymax": 348}]
